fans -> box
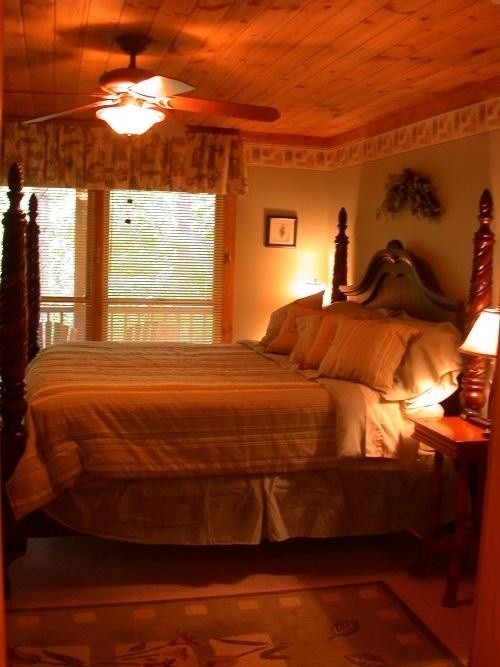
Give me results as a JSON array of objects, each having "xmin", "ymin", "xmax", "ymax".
[{"xmin": 3, "ymin": 33, "xmax": 282, "ymax": 126}]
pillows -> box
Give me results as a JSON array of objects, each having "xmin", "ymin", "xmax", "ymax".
[{"xmin": 254, "ymin": 289, "xmax": 465, "ymax": 408}]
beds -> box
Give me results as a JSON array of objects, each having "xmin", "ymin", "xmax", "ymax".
[{"xmin": 1, "ymin": 160, "xmax": 494, "ymax": 560}]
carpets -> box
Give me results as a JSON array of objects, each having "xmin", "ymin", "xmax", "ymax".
[{"xmin": 6, "ymin": 580, "xmax": 466, "ymax": 666}]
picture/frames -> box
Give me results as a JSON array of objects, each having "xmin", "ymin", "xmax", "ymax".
[{"xmin": 265, "ymin": 214, "xmax": 298, "ymax": 248}]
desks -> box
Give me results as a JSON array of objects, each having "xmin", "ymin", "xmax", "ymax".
[{"xmin": 408, "ymin": 415, "xmax": 491, "ymax": 608}]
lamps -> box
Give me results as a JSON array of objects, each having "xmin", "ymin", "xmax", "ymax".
[
  {"xmin": 95, "ymin": 96, "xmax": 166, "ymax": 136},
  {"xmin": 456, "ymin": 307, "xmax": 500, "ymax": 429}
]
